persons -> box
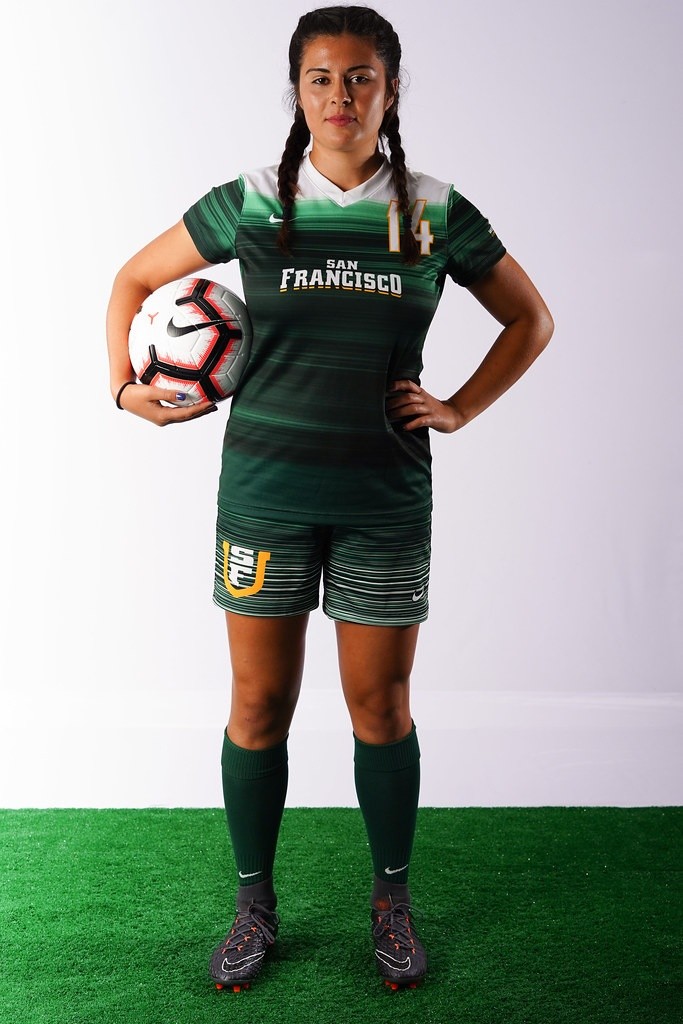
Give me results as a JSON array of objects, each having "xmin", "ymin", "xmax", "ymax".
[{"xmin": 105, "ymin": 6, "xmax": 553, "ymax": 996}]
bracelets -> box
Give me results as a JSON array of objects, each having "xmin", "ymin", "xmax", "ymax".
[{"xmin": 116, "ymin": 381, "xmax": 137, "ymax": 410}]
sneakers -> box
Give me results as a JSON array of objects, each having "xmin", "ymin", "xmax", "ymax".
[
  {"xmin": 369, "ymin": 900, "xmax": 430, "ymax": 991},
  {"xmin": 209, "ymin": 908, "xmax": 280, "ymax": 994}
]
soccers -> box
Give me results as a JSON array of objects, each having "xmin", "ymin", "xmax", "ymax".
[{"xmin": 127, "ymin": 277, "xmax": 254, "ymax": 408}]
